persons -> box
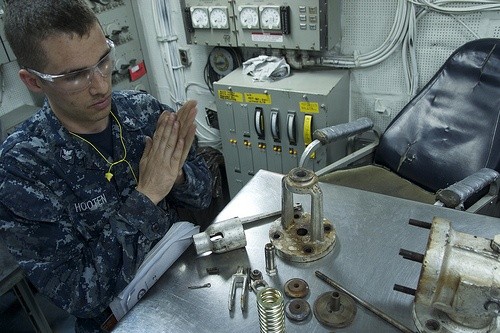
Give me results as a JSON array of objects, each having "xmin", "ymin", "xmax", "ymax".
[{"xmin": 0, "ymin": 0, "xmax": 214, "ymax": 333}]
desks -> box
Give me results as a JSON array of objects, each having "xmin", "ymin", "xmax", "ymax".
[{"xmin": 106, "ymin": 169, "xmax": 500, "ymax": 333}]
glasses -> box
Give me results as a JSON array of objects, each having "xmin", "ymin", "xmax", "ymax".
[{"xmin": 27, "ymin": 37, "xmax": 116, "ymax": 93}]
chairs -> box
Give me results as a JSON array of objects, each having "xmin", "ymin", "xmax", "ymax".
[{"xmin": 298, "ymin": 38, "xmax": 500, "ymax": 214}]
